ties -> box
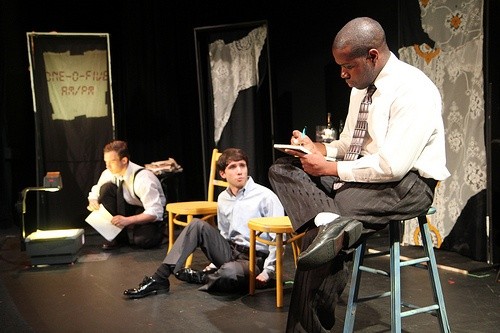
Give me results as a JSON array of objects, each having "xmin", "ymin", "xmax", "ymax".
[
  {"xmin": 117, "ymin": 179, "xmax": 124, "ymax": 215},
  {"xmin": 334, "ymin": 84, "xmax": 377, "ymax": 190}
]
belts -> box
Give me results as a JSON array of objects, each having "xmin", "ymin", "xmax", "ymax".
[{"xmin": 232, "ymin": 244, "xmax": 269, "ymax": 260}]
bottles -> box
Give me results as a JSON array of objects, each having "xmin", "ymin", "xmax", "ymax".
[
  {"xmin": 325, "ymin": 113, "xmax": 336, "ymax": 141},
  {"xmin": 338, "ymin": 120, "xmax": 344, "ymax": 137}
]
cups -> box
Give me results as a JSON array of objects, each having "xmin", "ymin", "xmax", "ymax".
[{"xmin": 315, "ymin": 125, "xmax": 326, "ymax": 142}]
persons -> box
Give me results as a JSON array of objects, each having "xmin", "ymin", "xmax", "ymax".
[
  {"xmin": 123, "ymin": 148, "xmax": 286, "ymax": 299},
  {"xmin": 85, "ymin": 142, "xmax": 165, "ymax": 251},
  {"xmin": 266, "ymin": 17, "xmax": 451, "ymax": 333}
]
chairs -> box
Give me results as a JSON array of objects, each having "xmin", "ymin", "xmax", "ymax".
[
  {"xmin": 247, "ymin": 216, "xmax": 305, "ymax": 308},
  {"xmin": 166, "ymin": 148, "xmax": 229, "ymax": 269}
]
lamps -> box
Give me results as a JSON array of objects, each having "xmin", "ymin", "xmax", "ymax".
[{"xmin": 20, "ymin": 171, "xmax": 63, "ymax": 250}]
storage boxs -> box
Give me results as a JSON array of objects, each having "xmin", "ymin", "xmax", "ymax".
[{"xmin": 25, "ymin": 229, "xmax": 85, "ymax": 256}]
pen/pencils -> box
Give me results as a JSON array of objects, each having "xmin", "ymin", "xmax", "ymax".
[{"xmin": 300, "ymin": 126, "xmax": 307, "ymax": 146}]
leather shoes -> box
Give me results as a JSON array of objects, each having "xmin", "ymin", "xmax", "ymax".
[
  {"xmin": 122, "ymin": 275, "xmax": 170, "ymax": 297},
  {"xmin": 175, "ymin": 268, "xmax": 204, "ymax": 283},
  {"xmin": 297, "ymin": 217, "xmax": 363, "ymax": 263},
  {"xmin": 102, "ymin": 241, "xmax": 122, "ymax": 250}
]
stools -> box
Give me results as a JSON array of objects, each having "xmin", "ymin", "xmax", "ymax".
[{"xmin": 344, "ymin": 207, "xmax": 451, "ymax": 333}]
